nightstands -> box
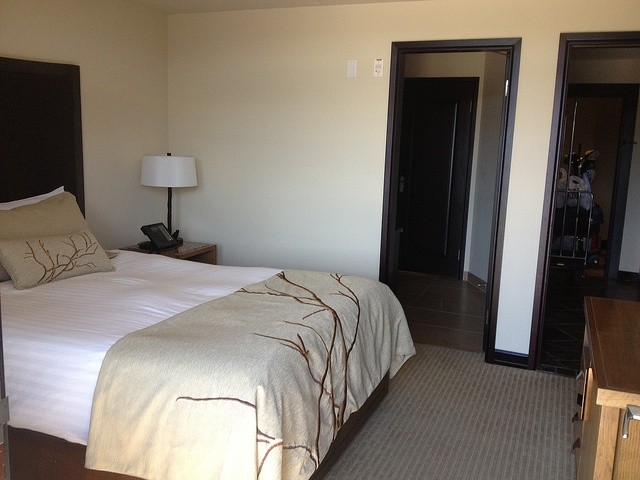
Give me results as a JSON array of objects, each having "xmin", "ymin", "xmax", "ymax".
[{"xmin": 118, "ymin": 237, "xmax": 217, "ymax": 265}]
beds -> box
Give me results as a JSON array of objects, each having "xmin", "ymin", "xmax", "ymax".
[{"xmin": 1, "ymin": 54, "xmax": 416, "ymax": 480}]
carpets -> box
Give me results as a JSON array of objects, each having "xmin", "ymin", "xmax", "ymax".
[{"xmin": 311, "ymin": 339, "xmax": 570, "ymax": 480}]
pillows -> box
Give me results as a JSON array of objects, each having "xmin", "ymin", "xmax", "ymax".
[
  {"xmin": 1, "ymin": 191, "xmax": 121, "ymax": 282},
  {"xmin": 2, "ymin": 230, "xmax": 117, "ymax": 290}
]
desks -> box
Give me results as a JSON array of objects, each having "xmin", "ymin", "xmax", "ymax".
[{"xmin": 573, "ymin": 298, "xmax": 640, "ymax": 478}]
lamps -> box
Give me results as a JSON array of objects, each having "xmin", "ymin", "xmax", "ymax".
[{"xmin": 141, "ymin": 152, "xmax": 198, "ymax": 239}]
